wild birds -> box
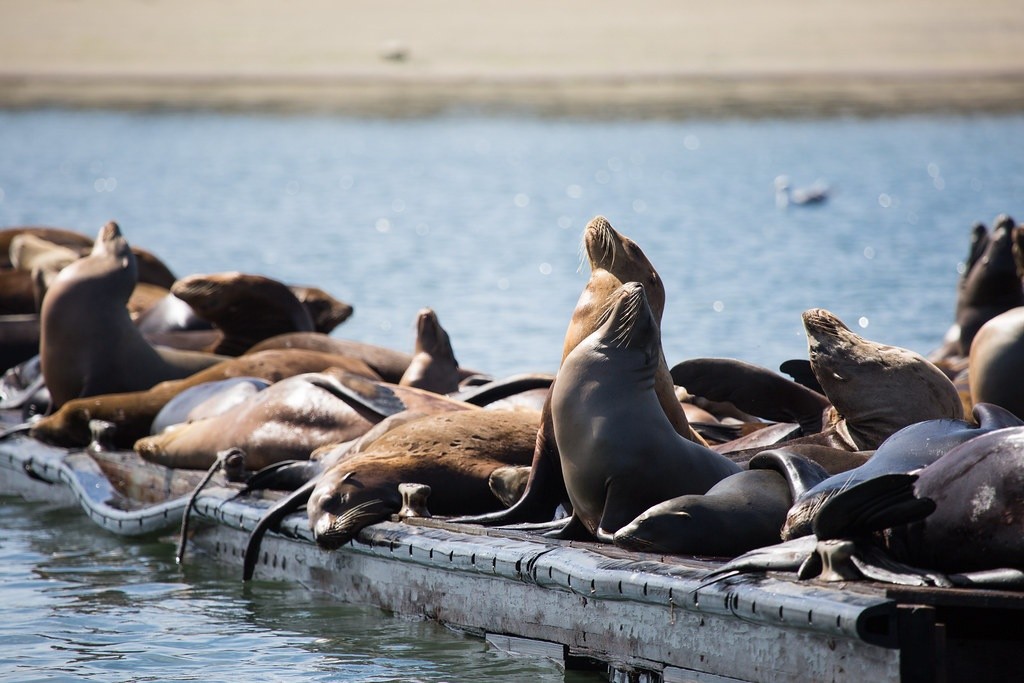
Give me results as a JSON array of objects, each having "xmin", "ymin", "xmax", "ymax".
[{"xmin": 775, "ymin": 177, "xmax": 830, "ymax": 210}]
